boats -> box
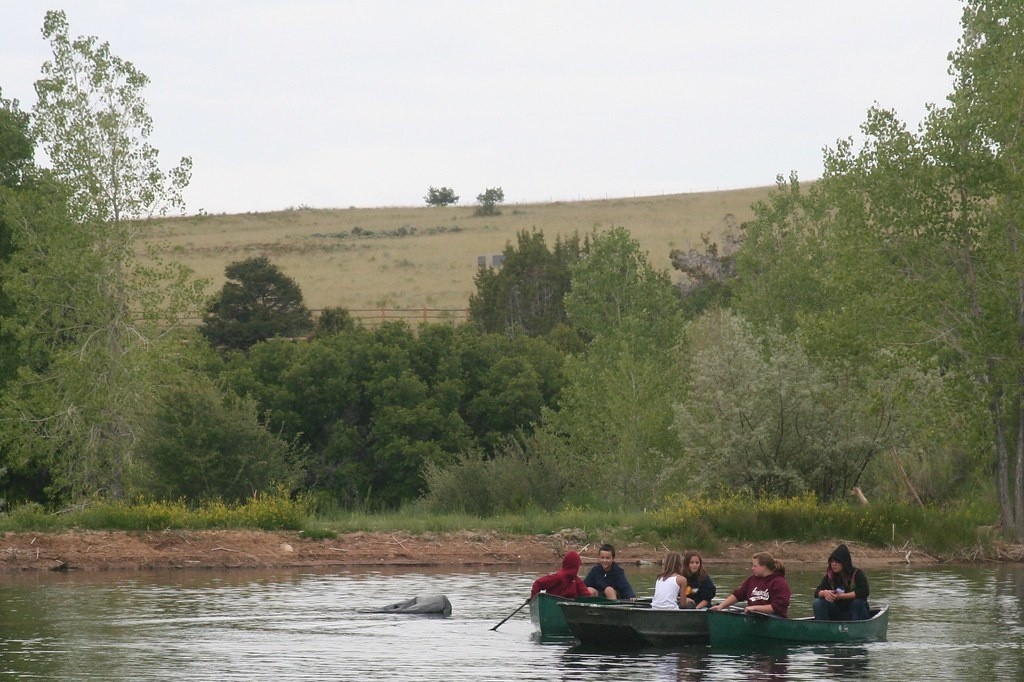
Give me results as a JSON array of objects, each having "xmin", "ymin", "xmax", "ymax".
[
  {"xmin": 527, "ymin": 591, "xmax": 657, "ymax": 643},
  {"xmin": 555, "ymin": 600, "xmax": 746, "ymax": 650},
  {"xmin": 705, "ymin": 601, "xmax": 890, "ymax": 654}
]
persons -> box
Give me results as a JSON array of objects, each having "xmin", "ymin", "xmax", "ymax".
[
  {"xmin": 813, "ymin": 544, "xmax": 870, "ymax": 621},
  {"xmin": 709, "ymin": 552, "xmax": 791, "ymax": 619},
  {"xmin": 649, "ymin": 551, "xmax": 695, "ymax": 609},
  {"xmin": 582, "ymin": 544, "xmax": 637, "ymax": 602},
  {"xmin": 677, "ymin": 551, "xmax": 716, "ymax": 609},
  {"xmin": 526, "ymin": 551, "xmax": 591, "ymax": 602}
]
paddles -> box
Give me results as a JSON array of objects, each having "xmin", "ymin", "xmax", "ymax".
[
  {"xmin": 601, "ymin": 600, "xmax": 652, "ymax": 605},
  {"xmin": 491, "ymin": 543, "xmax": 590, "ymax": 630},
  {"xmin": 728, "ymin": 604, "xmax": 782, "ymax": 619}
]
